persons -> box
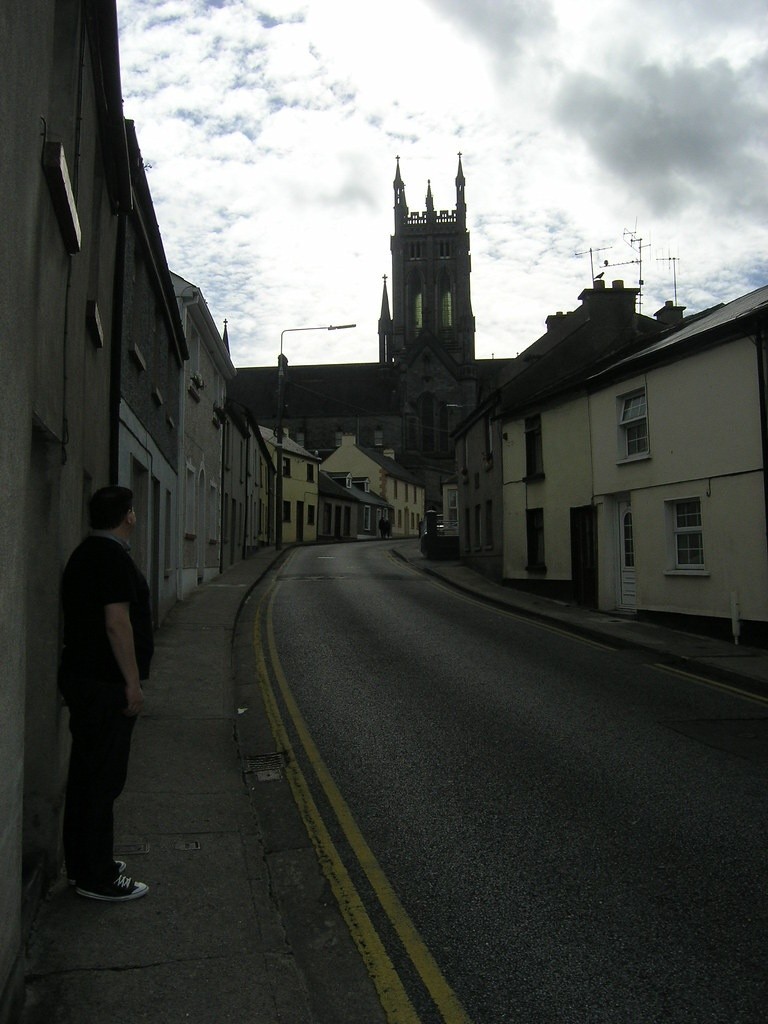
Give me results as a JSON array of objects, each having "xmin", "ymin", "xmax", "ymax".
[
  {"xmin": 62, "ymin": 485, "xmax": 155, "ymax": 901},
  {"xmin": 379, "ymin": 517, "xmax": 390, "ymax": 538}
]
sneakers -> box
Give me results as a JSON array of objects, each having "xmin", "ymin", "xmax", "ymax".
[
  {"xmin": 69, "ymin": 861, "xmax": 127, "ymax": 885},
  {"xmin": 76, "ymin": 875, "xmax": 148, "ymax": 902}
]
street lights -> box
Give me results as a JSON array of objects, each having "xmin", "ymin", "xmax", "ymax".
[{"xmin": 275, "ymin": 323, "xmax": 357, "ymax": 551}]
